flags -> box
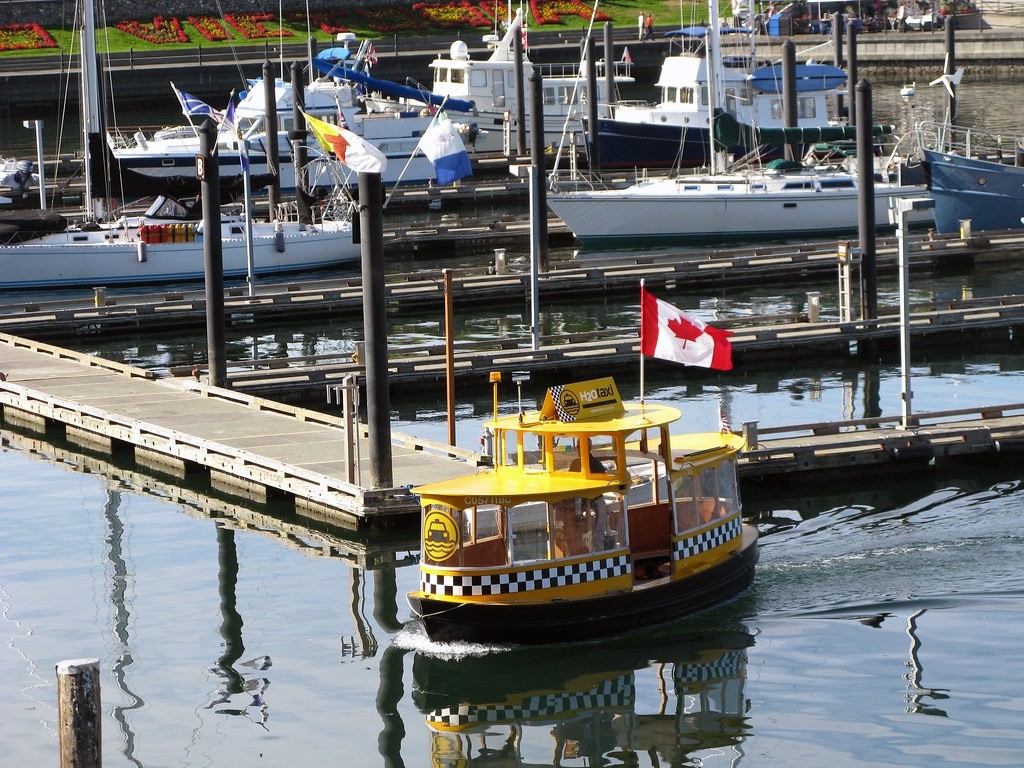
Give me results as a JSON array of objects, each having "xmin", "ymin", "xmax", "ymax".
[
  {"xmin": 417, "ymin": 108, "xmax": 473, "ymax": 188},
  {"xmin": 303, "ymin": 113, "xmax": 387, "ymax": 173},
  {"xmin": 640, "ymin": 286, "xmax": 734, "ymax": 370},
  {"xmin": 174, "ymin": 86, "xmax": 236, "ymax": 132}
]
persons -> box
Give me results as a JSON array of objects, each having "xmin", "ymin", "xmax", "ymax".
[
  {"xmin": 896, "ymin": 3, "xmax": 908, "ymax": 34},
  {"xmin": 469, "ymin": 123, "xmax": 478, "ymax": 147},
  {"xmin": 638, "ymin": 11, "xmax": 655, "ymax": 41},
  {"xmin": 568, "ymin": 436, "xmax": 616, "ymax": 533},
  {"xmin": 0, "ymin": 160, "xmax": 39, "ymax": 210}
]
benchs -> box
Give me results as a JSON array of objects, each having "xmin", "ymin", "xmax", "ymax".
[{"xmin": 628, "ymin": 544, "xmax": 671, "ymax": 583}]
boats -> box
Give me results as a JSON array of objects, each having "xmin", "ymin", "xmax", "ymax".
[
  {"xmin": 406, "ymin": 278, "xmax": 759, "ymax": 639},
  {"xmin": 0, "ymin": 0, "xmax": 1024, "ymax": 289}
]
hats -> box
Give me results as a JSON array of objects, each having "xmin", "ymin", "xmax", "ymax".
[{"xmin": 577, "ymin": 438, "xmax": 592, "ymax": 450}]
